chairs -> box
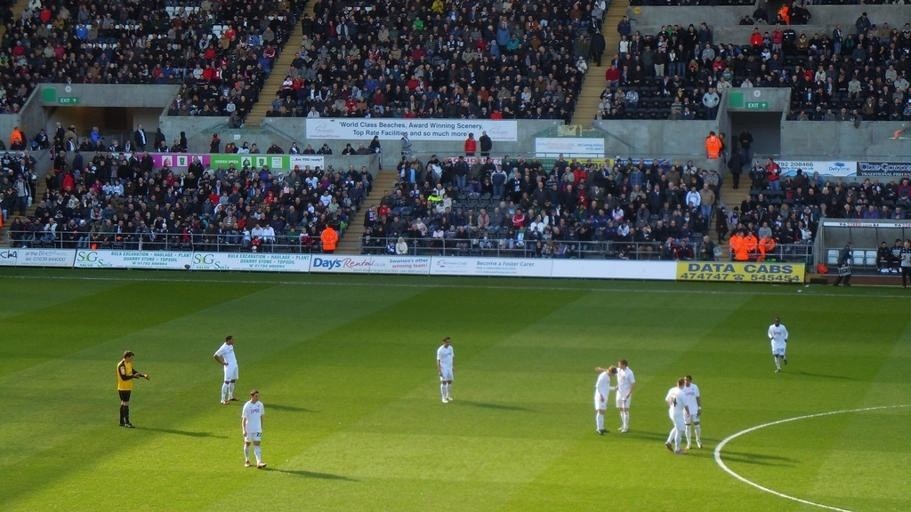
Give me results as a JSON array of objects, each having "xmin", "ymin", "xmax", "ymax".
[{"xmin": 603, "ymin": 44, "xmax": 862, "ymax": 120}]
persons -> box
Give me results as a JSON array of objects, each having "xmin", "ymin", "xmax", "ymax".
[
  {"xmin": 681, "ymin": 374, "xmax": 705, "ymax": 451},
  {"xmin": 115, "ymin": 347, "xmax": 151, "ymax": 428},
  {"xmin": 768, "ymin": 316, "xmax": 791, "ymax": 373},
  {"xmin": 595, "ymin": 358, "xmax": 637, "ymax": 433},
  {"xmin": 241, "ymin": 388, "xmax": 269, "ymax": 468},
  {"xmin": 214, "ymin": 335, "xmax": 242, "ymax": 405},
  {"xmin": 665, "ymin": 377, "xmax": 690, "ymax": 455},
  {"xmin": 436, "ymin": 335, "xmax": 456, "ymax": 405},
  {"xmin": 593, "ymin": 365, "xmax": 621, "ymax": 436},
  {"xmin": 0, "ymin": 1, "xmax": 911, "ymax": 289}
]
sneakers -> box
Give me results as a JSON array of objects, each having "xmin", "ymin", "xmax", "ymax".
[
  {"xmin": 245, "ymin": 461, "xmax": 250, "ymax": 467},
  {"xmin": 775, "ymin": 368, "xmax": 782, "ymax": 373},
  {"xmin": 665, "ymin": 441, "xmax": 702, "ymax": 453},
  {"xmin": 618, "ymin": 426, "xmax": 629, "ymax": 432},
  {"xmin": 120, "ymin": 423, "xmax": 134, "ymax": 428},
  {"xmin": 230, "ymin": 398, "xmax": 239, "ymax": 401},
  {"xmin": 595, "ymin": 428, "xmax": 608, "ymax": 435},
  {"xmin": 784, "ymin": 358, "xmax": 787, "ymax": 365},
  {"xmin": 442, "ymin": 397, "xmax": 454, "ymax": 403},
  {"xmin": 221, "ymin": 400, "xmax": 229, "ymax": 404},
  {"xmin": 257, "ymin": 462, "xmax": 266, "ymax": 468}
]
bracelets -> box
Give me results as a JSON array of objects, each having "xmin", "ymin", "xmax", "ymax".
[{"xmin": 698, "ymin": 406, "xmax": 703, "ymax": 409}]
